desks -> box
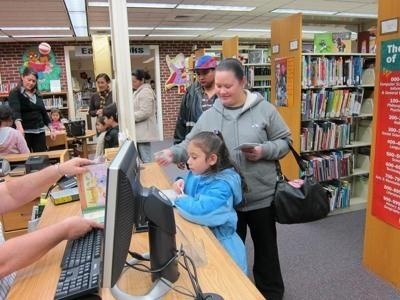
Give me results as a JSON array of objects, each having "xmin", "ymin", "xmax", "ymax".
[
  {"xmin": 5, "ymin": 162, "xmax": 266, "ymax": 299},
  {"xmin": 44, "ymin": 128, "xmax": 96, "ymax": 158}
]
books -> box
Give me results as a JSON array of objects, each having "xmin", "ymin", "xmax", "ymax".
[
  {"xmin": 231, "ymin": 142, "xmax": 263, "ymax": 150},
  {"xmin": 75, "ymin": 157, "xmax": 107, "ymax": 220},
  {"xmin": 205, "ymin": 29, "xmax": 380, "ymax": 214},
  {"xmin": 47, "ymin": 185, "xmax": 80, "ymax": 206}
]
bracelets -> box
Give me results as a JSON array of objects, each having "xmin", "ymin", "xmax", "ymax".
[{"xmin": 55, "ymin": 161, "xmax": 63, "ymax": 178}]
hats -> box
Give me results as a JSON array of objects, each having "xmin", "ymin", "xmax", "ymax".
[{"xmin": 189, "ymin": 56, "xmax": 217, "ymax": 70}]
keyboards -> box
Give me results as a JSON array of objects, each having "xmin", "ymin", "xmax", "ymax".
[{"xmin": 54, "ymin": 226, "xmax": 104, "ymax": 300}]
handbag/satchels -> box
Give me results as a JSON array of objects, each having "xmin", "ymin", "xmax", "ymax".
[{"xmin": 274, "ymin": 175, "xmax": 329, "ymax": 224}]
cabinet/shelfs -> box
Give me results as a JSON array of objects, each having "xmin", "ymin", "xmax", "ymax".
[
  {"xmin": 271, "ymin": 11, "xmax": 370, "ymax": 216},
  {"xmin": 189, "ymin": 35, "xmax": 272, "ymax": 105},
  {"xmin": 1, "ymin": 91, "xmax": 69, "ymax": 124},
  {"xmin": 2, "ymin": 201, "xmax": 42, "ymax": 238},
  {"xmin": 361, "ymin": 1, "xmax": 400, "ymax": 292}
]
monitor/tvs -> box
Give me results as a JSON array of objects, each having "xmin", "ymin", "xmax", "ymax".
[{"xmin": 101, "ymin": 139, "xmax": 180, "ymax": 300}]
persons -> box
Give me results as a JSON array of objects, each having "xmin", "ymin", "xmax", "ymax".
[
  {"xmin": 151, "ymin": 57, "xmax": 295, "ymax": 300},
  {"xmin": 173, "ymin": 54, "xmax": 219, "ymax": 146},
  {"xmin": 1, "ymin": 155, "xmax": 106, "ymax": 300},
  {"xmin": 172, "ymin": 128, "xmax": 251, "ymax": 282},
  {"xmin": 1, "ymin": 65, "xmax": 157, "ymax": 165}
]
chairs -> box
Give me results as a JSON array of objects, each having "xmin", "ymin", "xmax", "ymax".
[{"xmin": 44, "ymin": 133, "xmax": 67, "ymax": 151}]
